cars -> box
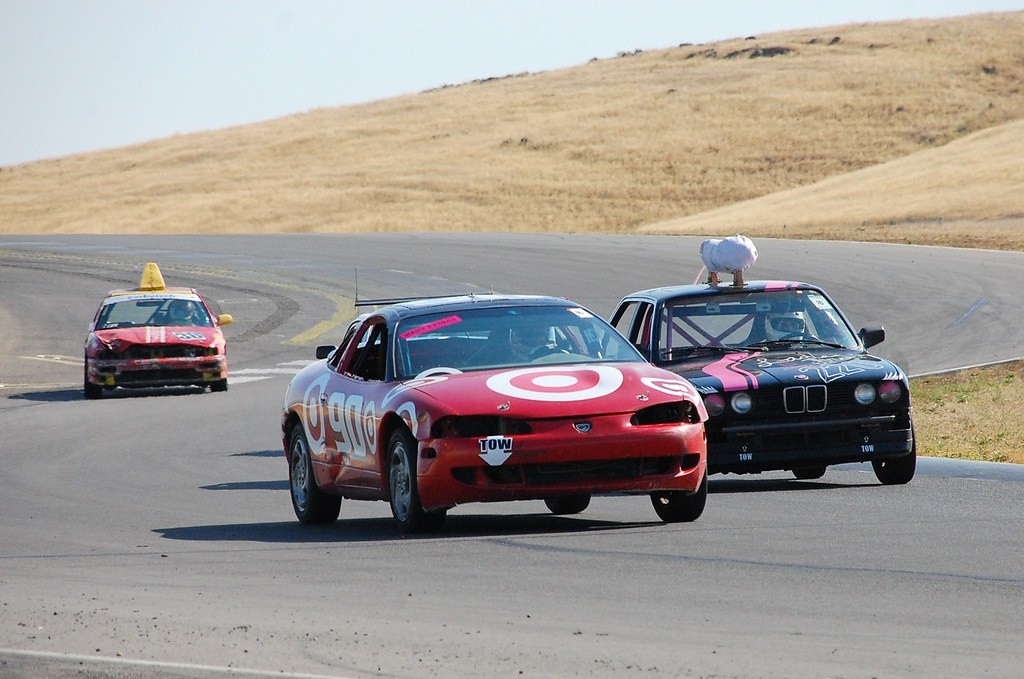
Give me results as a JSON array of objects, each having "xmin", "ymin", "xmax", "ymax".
[
  {"xmin": 601, "ymin": 233, "xmax": 917, "ymax": 495},
  {"xmin": 84, "ymin": 262, "xmax": 233, "ymax": 398},
  {"xmin": 281, "ymin": 266, "xmax": 710, "ymax": 535}
]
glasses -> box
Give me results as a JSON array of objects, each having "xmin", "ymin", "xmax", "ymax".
[{"xmin": 774, "ymin": 319, "xmax": 805, "ymax": 332}]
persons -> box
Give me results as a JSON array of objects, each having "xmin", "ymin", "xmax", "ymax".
[
  {"xmin": 496, "ymin": 325, "xmax": 559, "ymax": 364},
  {"xmin": 167, "ymin": 300, "xmax": 196, "ymax": 323},
  {"xmin": 759, "ymin": 312, "xmax": 807, "ymax": 342}
]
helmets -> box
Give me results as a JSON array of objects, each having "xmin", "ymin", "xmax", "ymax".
[
  {"xmin": 509, "ymin": 322, "xmax": 557, "ymax": 360},
  {"xmin": 169, "ymin": 300, "xmax": 194, "ymax": 321},
  {"xmin": 766, "ymin": 309, "xmax": 805, "ymax": 345}
]
